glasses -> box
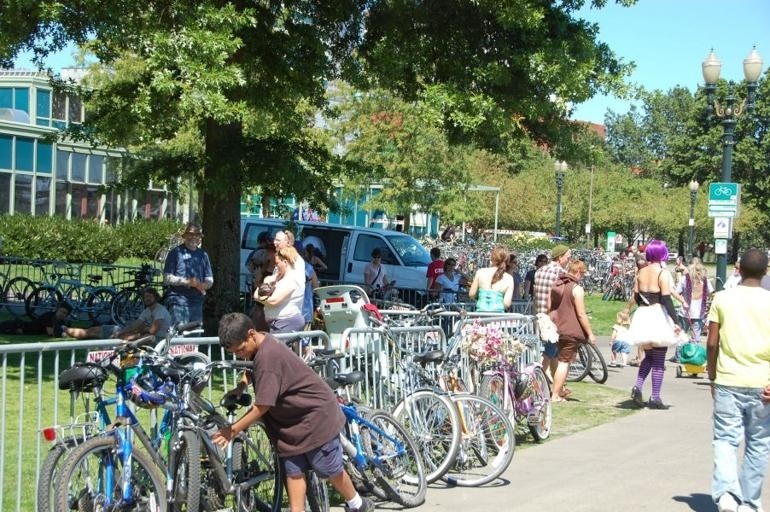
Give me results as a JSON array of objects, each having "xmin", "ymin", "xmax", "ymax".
[{"xmin": 373, "ymin": 256, "xmax": 381, "ymax": 259}]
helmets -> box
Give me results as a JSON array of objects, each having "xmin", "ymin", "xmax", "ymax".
[{"xmin": 123, "ymin": 367, "xmax": 178, "ymax": 408}]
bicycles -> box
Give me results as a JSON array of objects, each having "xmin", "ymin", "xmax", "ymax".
[
  {"xmin": 0, "ymin": 255, "xmax": 167, "ymax": 327},
  {"xmin": 565, "ymin": 311, "xmax": 609, "ymax": 385},
  {"xmin": 411, "ymin": 217, "xmax": 636, "ymax": 302},
  {"xmin": 37, "ymin": 302, "xmax": 554, "ymax": 512}
]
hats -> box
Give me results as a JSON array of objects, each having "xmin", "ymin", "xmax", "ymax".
[{"xmin": 551, "ymin": 244, "xmax": 568, "ymax": 259}]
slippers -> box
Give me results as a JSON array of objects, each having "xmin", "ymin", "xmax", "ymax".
[
  {"xmin": 559, "ymin": 389, "xmax": 571, "ymax": 396},
  {"xmin": 551, "ymin": 398, "xmax": 567, "ymax": 403}
]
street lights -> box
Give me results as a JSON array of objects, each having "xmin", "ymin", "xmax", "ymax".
[
  {"xmin": 700, "ymin": 42, "xmax": 765, "ymax": 295},
  {"xmin": 687, "ymin": 178, "xmax": 700, "ymax": 264},
  {"xmin": 552, "ymin": 158, "xmax": 570, "ymax": 238}
]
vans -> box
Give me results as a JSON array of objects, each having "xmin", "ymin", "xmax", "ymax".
[{"xmin": 237, "ymin": 220, "xmax": 433, "ymax": 303}]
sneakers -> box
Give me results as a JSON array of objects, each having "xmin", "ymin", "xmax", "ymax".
[
  {"xmin": 739, "ymin": 504, "xmax": 764, "ymax": 512},
  {"xmin": 716, "ymin": 493, "xmax": 738, "ymax": 512},
  {"xmin": 345, "ymin": 497, "xmax": 374, "ymax": 511}
]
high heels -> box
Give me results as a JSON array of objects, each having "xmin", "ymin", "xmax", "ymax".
[
  {"xmin": 649, "ymin": 397, "xmax": 668, "ymax": 409},
  {"xmin": 631, "ymin": 386, "xmax": 642, "ymax": 407}
]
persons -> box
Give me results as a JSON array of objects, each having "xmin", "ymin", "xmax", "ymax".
[
  {"xmin": 761, "ymin": 273, "xmax": 770, "ymax": 290},
  {"xmin": 212, "ymin": 311, "xmax": 375, "ymax": 510},
  {"xmin": 426, "ymin": 244, "xmax": 596, "ymax": 402},
  {"xmin": 112, "ymin": 227, "xmax": 328, "ymax": 356},
  {"xmin": 604, "ymin": 244, "xmax": 714, "ymax": 368},
  {"xmin": 365, "ymin": 244, "xmax": 392, "ymax": 299},
  {"xmin": 59, "ymin": 323, "xmax": 123, "ymax": 339},
  {"xmin": 722, "ymin": 257, "xmax": 742, "ymax": 290},
  {"xmin": 1, "ymin": 303, "xmax": 72, "ymax": 338},
  {"xmin": 706, "ymin": 249, "xmax": 770, "ymax": 512},
  {"xmin": 628, "ymin": 239, "xmax": 681, "ymax": 409}
]
